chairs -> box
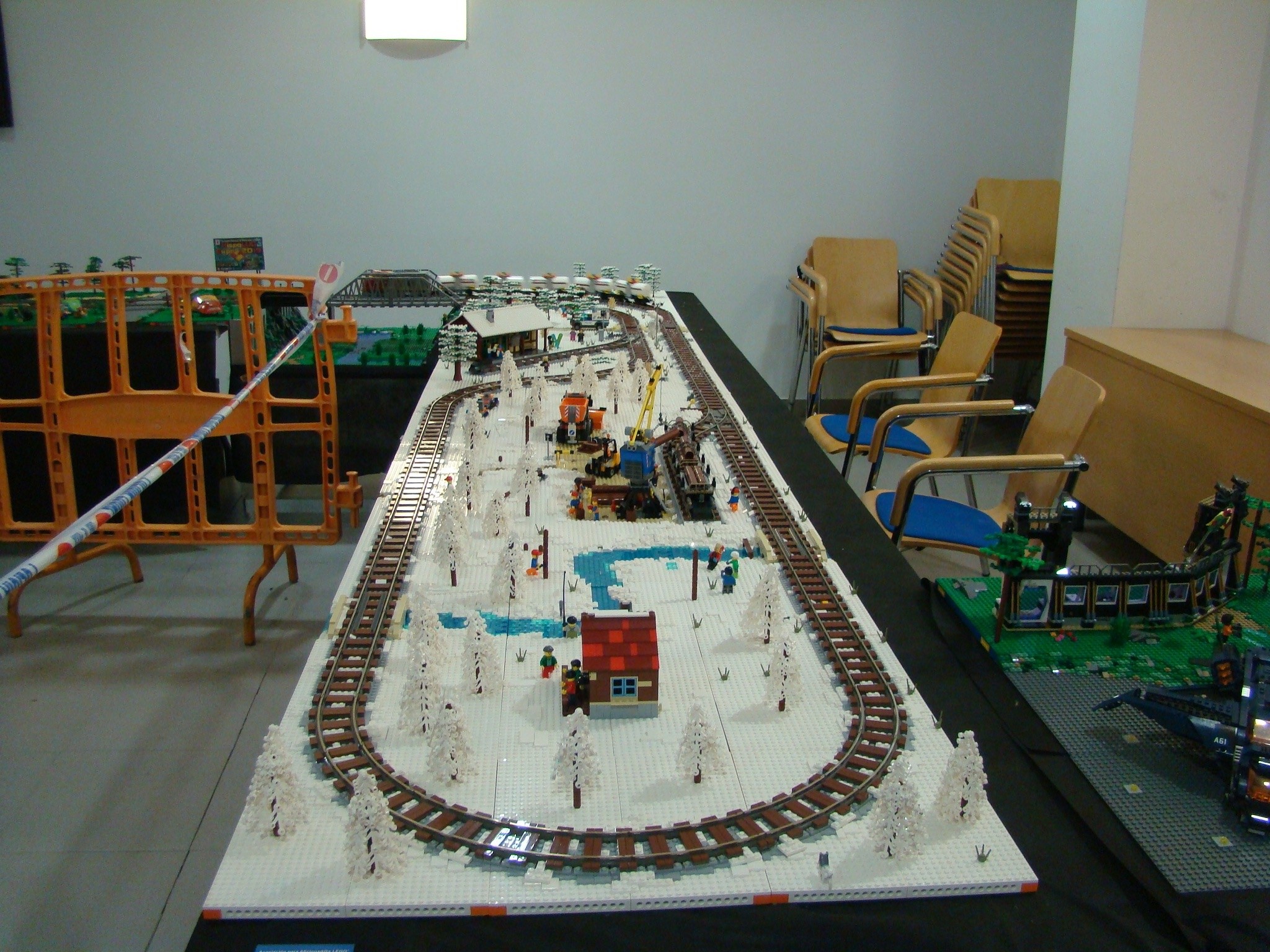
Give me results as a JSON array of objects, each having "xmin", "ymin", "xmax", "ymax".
[{"xmin": 785, "ymin": 177, "xmax": 1108, "ymax": 578}]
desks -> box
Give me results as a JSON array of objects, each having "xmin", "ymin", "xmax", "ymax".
[
  {"xmin": 230, "ymin": 374, "xmax": 432, "ymax": 517},
  {"xmin": 0, "ymin": 321, "xmax": 229, "ymax": 524},
  {"xmin": 1062, "ymin": 325, "xmax": 1270, "ymax": 578}
]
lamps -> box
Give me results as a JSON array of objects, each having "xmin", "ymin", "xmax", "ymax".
[{"xmin": 364, "ymin": 0, "xmax": 467, "ymax": 41}]
